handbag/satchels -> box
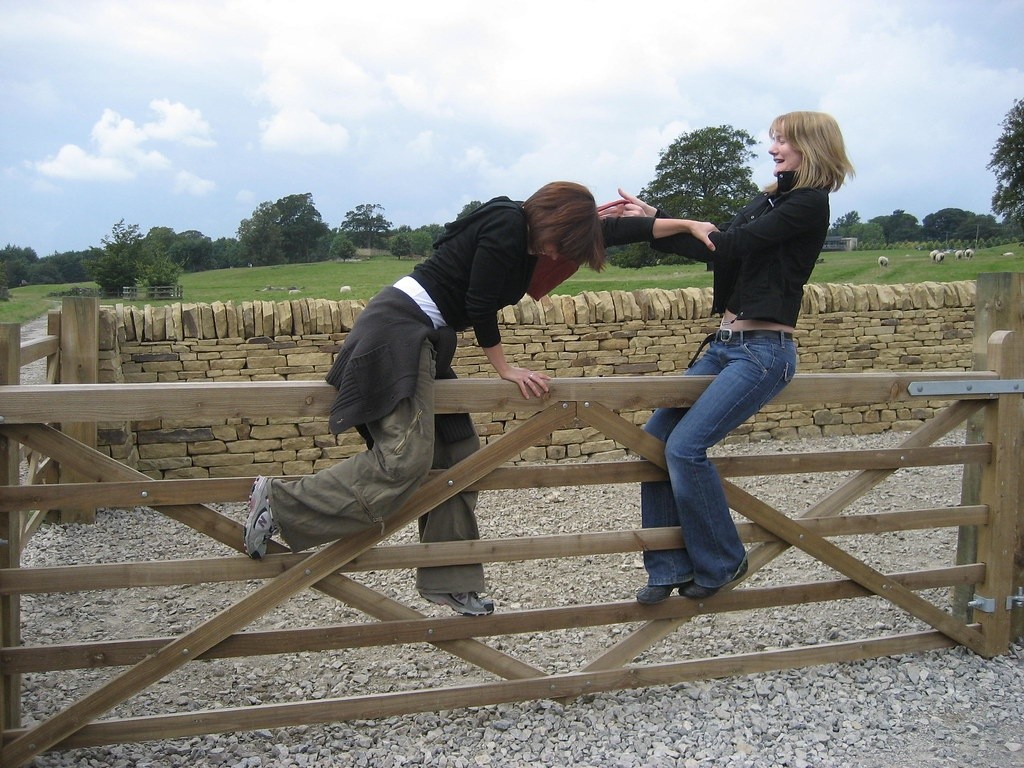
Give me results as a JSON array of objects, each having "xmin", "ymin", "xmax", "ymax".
[{"xmin": 525, "ymin": 200, "xmax": 631, "ymax": 302}]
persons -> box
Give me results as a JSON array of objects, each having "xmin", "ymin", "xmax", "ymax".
[
  {"xmin": 616, "ymin": 112, "xmax": 855, "ymax": 604},
  {"xmin": 244, "ymin": 182, "xmax": 722, "ymax": 616}
]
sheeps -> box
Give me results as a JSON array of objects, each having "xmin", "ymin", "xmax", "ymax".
[
  {"xmin": 877, "ymin": 256, "xmax": 888, "ymax": 269},
  {"xmin": 929, "ymin": 249, "xmax": 973, "ymax": 263},
  {"xmin": 340, "ymin": 286, "xmax": 350, "ymax": 294},
  {"xmin": 288, "ymin": 290, "xmax": 299, "ymax": 295},
  {"xmin": 1001, "ymin": 252, "xmax": 1014, "ymax": 256}
]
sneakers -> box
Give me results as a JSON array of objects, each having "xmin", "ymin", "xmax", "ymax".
[
  {"xmin": 419, "ymin": 590, "xmax": 495, "ymax": 616},
  {"xmin": 678, "ymin": 560, "xmax": 749, "ymax": 599},
  {"xmin": 637, "ymin": 580, "xmax": 692, "ymax": 604},
  {"xmin": 243, "ymin": 474, "xmax": 281, "ymax": 561}
]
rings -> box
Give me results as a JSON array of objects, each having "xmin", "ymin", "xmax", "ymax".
[
  {"xmin": 526, "ymin": 379, "xmax": 530, "ymax": 384},
  {"xmin": 530, "ymin": 373, "xmax": 533, "ymax": 379}
]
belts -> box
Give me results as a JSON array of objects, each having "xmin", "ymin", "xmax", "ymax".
[{"xmin": 688, "ymin": 331, "xmax": 792, "ymax": 368}]
